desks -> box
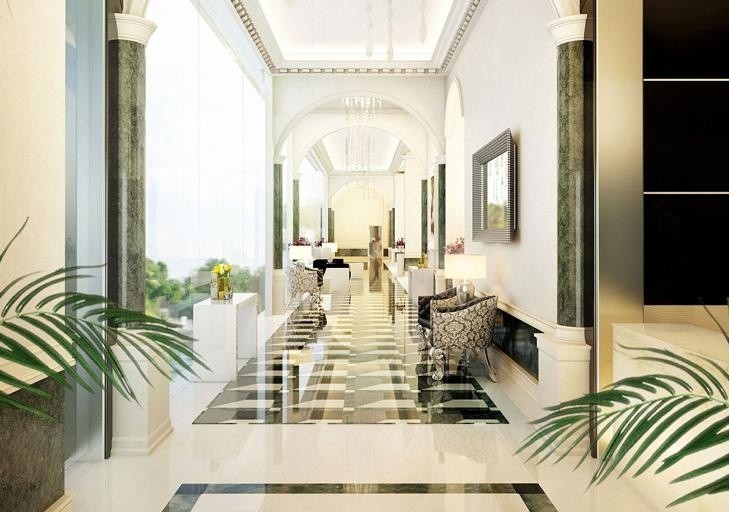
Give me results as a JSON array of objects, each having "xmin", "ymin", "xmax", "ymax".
[
  {"xmin": 192, "ymin": 293, "xmax": 257, "ymax": 383},
  {"xmin": 407, "ymin": 266, "xmax": 433, "ymax": 301}
]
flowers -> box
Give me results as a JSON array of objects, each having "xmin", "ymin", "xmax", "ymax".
[{"xmin": 213, "ymin": 263, "xmax": 232, "ymax": 289}]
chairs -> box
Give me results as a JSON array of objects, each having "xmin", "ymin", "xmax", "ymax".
[
  {"xmin": 285, "ymin": 258, "xmax": 351, "ymax": 308},
  {"xmin": 416, "ymin": 286, "xmax": 498, "ymax": 383}
]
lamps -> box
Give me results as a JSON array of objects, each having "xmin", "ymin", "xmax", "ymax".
[{"xmin": 444, "ymin": 253, "xmax": 487, "ymax": 305}]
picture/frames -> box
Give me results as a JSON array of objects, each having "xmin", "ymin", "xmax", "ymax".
[{"xmin": 471, "ymin": 127, "xmax": 516, "ymax": 244}]
